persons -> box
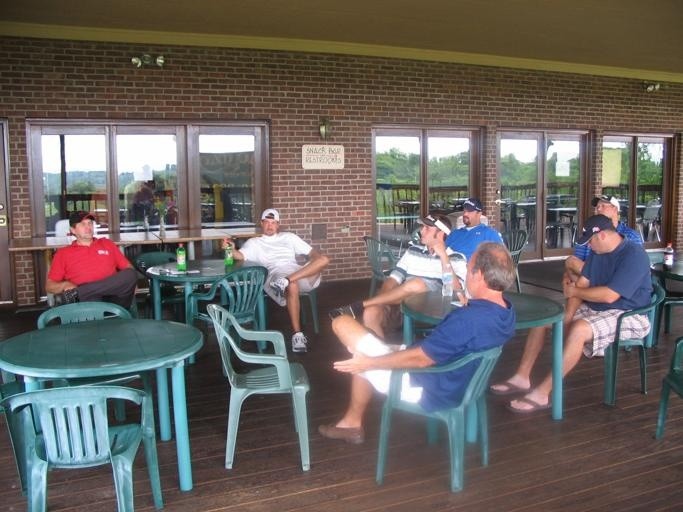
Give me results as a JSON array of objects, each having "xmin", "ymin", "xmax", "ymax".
[
  {"xmin": 490, "ymin": 213, "xmax": 655, "ymax": 410},
  {"xmin": 45, "ymin": 211, "xmax": 137, "ymax": 311},
  {"xmin": 564, "ymin": 195, "xmax": 643, "ymax": 282},
  {"xmin": 446, "ymin": 197, "xmax": 505, "ymax": 263},
  {"xmin": 329, "ymin": 213, "xmax": 467, "ymax": 339},
  {"xmin": 220, "ymin": 209, "xmax": 329, "ymax": 353},
  {"xmin": 318, "ymin": 241, "xmax": 516, "ymax": 446}
]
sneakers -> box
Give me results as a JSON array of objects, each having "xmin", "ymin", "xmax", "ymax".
[
  {"xmin": 268, "ymin": 276, "xmax": 288, "ymax": 296},
  {"xmin": 291, "ymin": 332, "xmax": 307, "ymax": 353},
  {"xmin": 317, "ymin": 420, "xmax": 365, "ymax": 444},
  {"xmin": 327, "ymin": 301, "xmax": 362, "ymax": 320}
]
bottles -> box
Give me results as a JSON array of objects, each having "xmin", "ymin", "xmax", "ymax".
[
  {"xmin": 662, "ymin": 242, "xmax": 674, "ymax": 270},
  {"xmin": 175, "ymin": 244, "xmax": 186, "ymax": 271},
  {"xmin": 157, "ymin": 215, "xmax": 166, "ymax": 239},
  {"xmin": 223, "ymin": 235, "xmax": 233, "ymax": 266},
  {"xmin": 142, "ymin": 216, "xmax": 149, "ymax": 240},
  {"xmin": 441, "ymin": 263, "xmax": 453, "ymax": 297}
]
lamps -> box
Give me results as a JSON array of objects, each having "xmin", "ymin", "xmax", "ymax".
[
  {"xmin": 128, "ymin": 53, "xmax": 165, "ymax": 72},
  {"xmin": 319, "ymin": 118, "xmax": 331, "ymax": 140},
  {"xmin": 641, "ymin": 80, "xmax": 670, "ymax": 93}
]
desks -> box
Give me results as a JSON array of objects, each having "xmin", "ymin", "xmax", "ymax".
[
  {"xmin": 5, "ymin": 318, "xmax": 206, "ymax": 498},
  {"xmin": 145, "ymin": 255, "xmax": 265, "ymax": 321},
  {"xmin": 648, "ymin": 261, "xmax": 682, "ymax": 344},
  {"xmin": 400, "ymin": 288, "xmax": 566, "ymax": 422}
]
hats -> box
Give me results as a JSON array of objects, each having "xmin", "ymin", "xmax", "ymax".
[
  {"xmin": 574, "ymin": 215, "xmax": 612, "ymax": 246},
  {"xmin": 69, "ymin": 210, "xmax": 95, "ymax": 226},
  {"xmin": 415, "ymin": 212, "xmax": 451, "ymax": 236},
  {"xmin": 462, "ymin": 198, "xmax": 482, "ymax": 212},
  {"xmin": 590, "ymin": 194, "xmax": 620, "ymax": 214},
  {"xmin": 261, "ymin": 208, "xmax": 280, "ymax": 222}
]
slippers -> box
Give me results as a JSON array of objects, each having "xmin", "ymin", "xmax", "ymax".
[
  {"xmin": 489, "ymin": 379, "xmax": 530, "ymax": 396},
  {"xmin": 503, "ymin": 394, "xmax": 553, "ymax": 416}
]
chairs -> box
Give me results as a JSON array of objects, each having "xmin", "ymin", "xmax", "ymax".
[
  {"xmin": 499, "ymin": 230, "xmax": 528, "ymax": 296},
  {"xmin": 137, "ymin": 251, "xmax": 178, "ymax": 275},
  {"xmin": 655, "ymin": 334, "xmax": 683, "ymax": 443},
  {"xmin": 601, "ymin": 279, "xmax": 666, "ymax": 404},
  {"xmin": 376, "ymin": 342, "xmax": 504, "ymax": 493},
  {"xmin": 397, "ymin": 194, "xmax": 664, "ymax": 248},
  {"xmin": 362, "ymin": 236, "xmax": 399, "ymax": 300},
  {"xmin": 204, "ymin": 302, "xmax": 314, "ymax": 474},
  {"xmin": 0, "ymin": 385, "xmax": 167, "ymax": 510},
  {"xmin": 37, "ymin": 300, "xmax": 132, "ymax": 329},
  {"xmin": 261, "ymin": 288, "xmax": 321, "ymax": 336},
  {"xmin": 183, "ymin": 266, "xmax": 270, "ymax": 367}
]
